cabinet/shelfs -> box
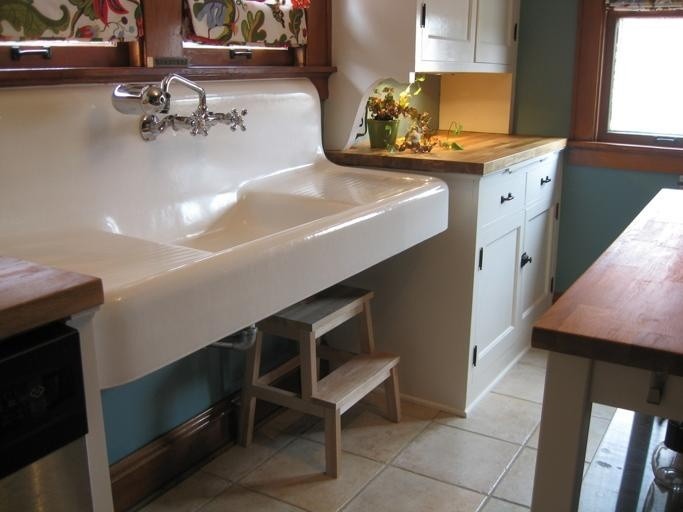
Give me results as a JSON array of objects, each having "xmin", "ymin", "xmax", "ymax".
[
  {"xmin": 325, "ymin": 129, "xmax": 572, "ymax": 422},
  {"xmin": 331, "ymin": 0, "xmax": 521, "ymax": 74}
]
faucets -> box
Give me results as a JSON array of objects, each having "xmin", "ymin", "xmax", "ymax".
[{"xmin": 158, "ymin": 74, "xmax": 206, "ymax": 114}]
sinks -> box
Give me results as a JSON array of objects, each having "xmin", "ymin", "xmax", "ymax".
[{"xmin": 100, "ymin": 190, "xmax": 359, "ymax": 253}]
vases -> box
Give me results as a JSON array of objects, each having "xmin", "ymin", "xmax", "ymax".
[{"xmin": 366, "ymin": 118, "xmax": 400, "ymax": 149}]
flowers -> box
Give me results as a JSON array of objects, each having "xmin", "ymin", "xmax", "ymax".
[{"xmin": 366, "ymin": 67, "xmax": 463, "ymax": 154}]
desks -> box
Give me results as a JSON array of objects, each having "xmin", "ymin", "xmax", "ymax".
[
  {"xmin": 525, "ymin": 182, "xmax": 683, "ymax": 509},
  {"xmin": 0, "ymin": 258, "xmax": 117, "ymax": 512}
]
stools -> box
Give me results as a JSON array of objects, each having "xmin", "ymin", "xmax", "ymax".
[{"xmin": 232, "ymin": 282, "xmax": 410, "ymax": 482}]
